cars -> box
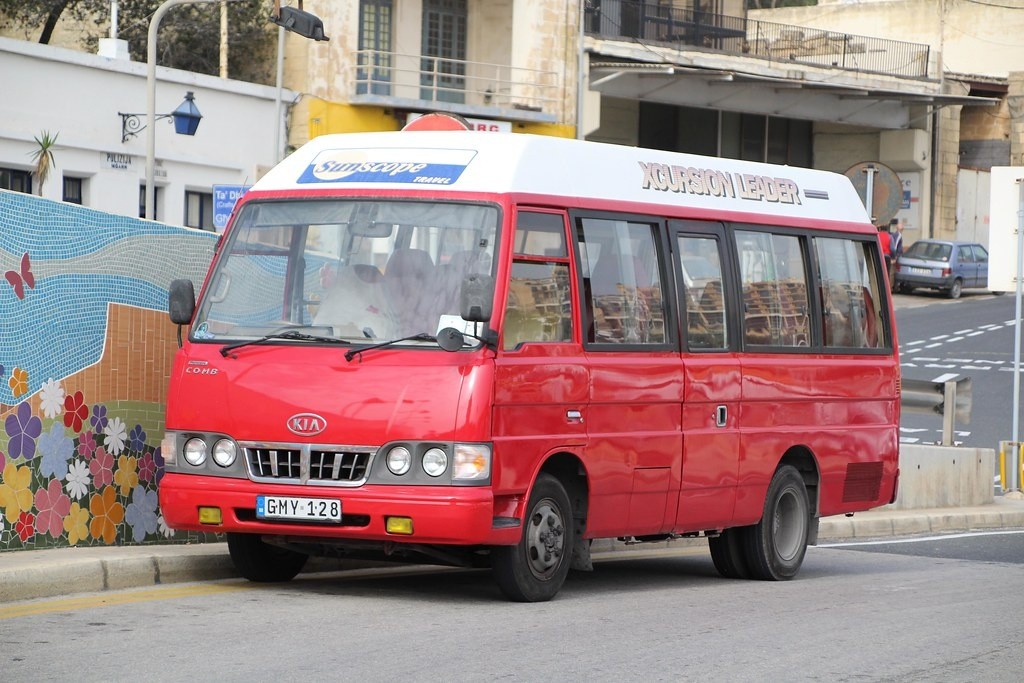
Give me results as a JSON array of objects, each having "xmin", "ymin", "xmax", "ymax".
[{"xmin": 893, "ymin": 240, "xmax": 1005, "ymax": 299}]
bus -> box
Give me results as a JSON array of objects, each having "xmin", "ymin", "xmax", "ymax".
[{"xmin": 168, "ymin": 131, "xmax": 902, "ymax": 603}]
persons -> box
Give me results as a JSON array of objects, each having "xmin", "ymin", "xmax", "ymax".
[{"xmin": 878, "ymin": 222, "xmax": 903, "ymax": 294}]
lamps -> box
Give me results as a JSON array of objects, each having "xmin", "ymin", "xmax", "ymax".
[{"xmin": 118, "ymin": 91, "xmax": 203, "ymax": 144}]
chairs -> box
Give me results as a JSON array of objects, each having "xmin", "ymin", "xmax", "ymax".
[
  {"xmin": 310, "ymin": 249, "xmax": 877, "ymax": 350},
  {"xmin": 932, "ymin": 247, "xmax": 944, "ymax": 257}
]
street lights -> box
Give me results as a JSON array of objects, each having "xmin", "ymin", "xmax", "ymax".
[{"xmin": 146, "ymin": 0, "xmax": 329, "ymax": 221}]
